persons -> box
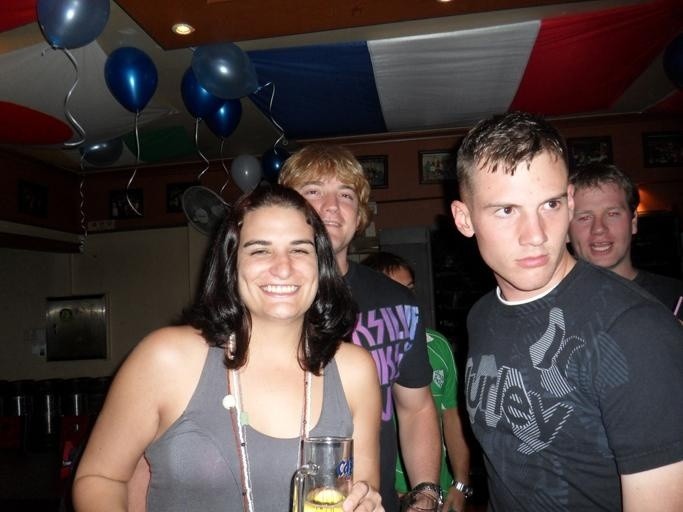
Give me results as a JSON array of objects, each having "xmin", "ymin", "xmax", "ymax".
[
  {"xmin": 126, "ymin": 141, "xmax": 441, "ymax": 512},
  {"xmin": 450, "ymin": 112, "xmax": 683, "ymax": 512},
  {"xmin": 362, "ymin": 247, "xmax": 471, "ymax": 512},
  {"xmin": 69, "ymin": 183, "xmax": 385, "ymax": 512},
  {"xmin": 567, "ymin": 163, "xmax": 683, "ymax": 323}
]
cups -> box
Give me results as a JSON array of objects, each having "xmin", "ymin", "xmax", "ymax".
[
  {"xmin": 289, "ymin": 434, "xmax": 355, "ymax": 512},
  {"xmin": 401, "ymin": 490, "xmax": 439, "ymax": 511}
]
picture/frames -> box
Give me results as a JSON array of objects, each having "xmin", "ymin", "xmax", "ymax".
[
  {"xmin": 166, "ymin": 180, "xmax": 202, "ymax": 214},
  {"xmin": 642, "ymin": 130, "xmax": 682, "ymax": 168},
  {"xmin": 17, "ymin": 180, "xmax": 48, "ymax": 219},
  {"xmin": 562, "ymin": 135, "xmax": 613, "ymax": 182},
  {"xmin": 108, "ymin": 188, "xmax": 144, "ymax": 220},
  {"xmin": 355, "ymin": 154, "xmax": 390, "ymax": 190},
  {"xmin": 417, "ymin": 148, "xmax": 453, "ymax": 186}
]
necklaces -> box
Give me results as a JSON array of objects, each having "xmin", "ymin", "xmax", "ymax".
[{"xmin": 226, "ymin": 334, "xmax": 312, "ymax": 512}]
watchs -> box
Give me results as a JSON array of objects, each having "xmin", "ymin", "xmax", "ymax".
[
  {"xmin": 411, "ymin": 482, "xmax": 443, "ymax": 505},
  {"xmin": 451, "ymin": 480, "xmax": 474, "ymax": 498}
]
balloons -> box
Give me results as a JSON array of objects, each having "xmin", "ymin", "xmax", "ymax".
[
  {"xmin": 37, "ymin": 0, "xmax": 111, "ymax": 50},
  {"xmin": 261, "ymin": 147, "xmax": 291, "ymax": 184},
  {"xmin": 191, "ymin": 41, "xmax": 258, "ymax": 100},
  {"xmin": 180, "ymin": 66, "xmax": 225, "ymax": 120},
  {"xmin": 231, "ymin": 154, "xmax": 262, "ymax": 194},
  {"xmin": 203, "ymin": 99, "xmax": 242, "ymax": 140},
  {"xmin": 104, "ymin": 46, "xmax": 158, "ymax": 113}
]
